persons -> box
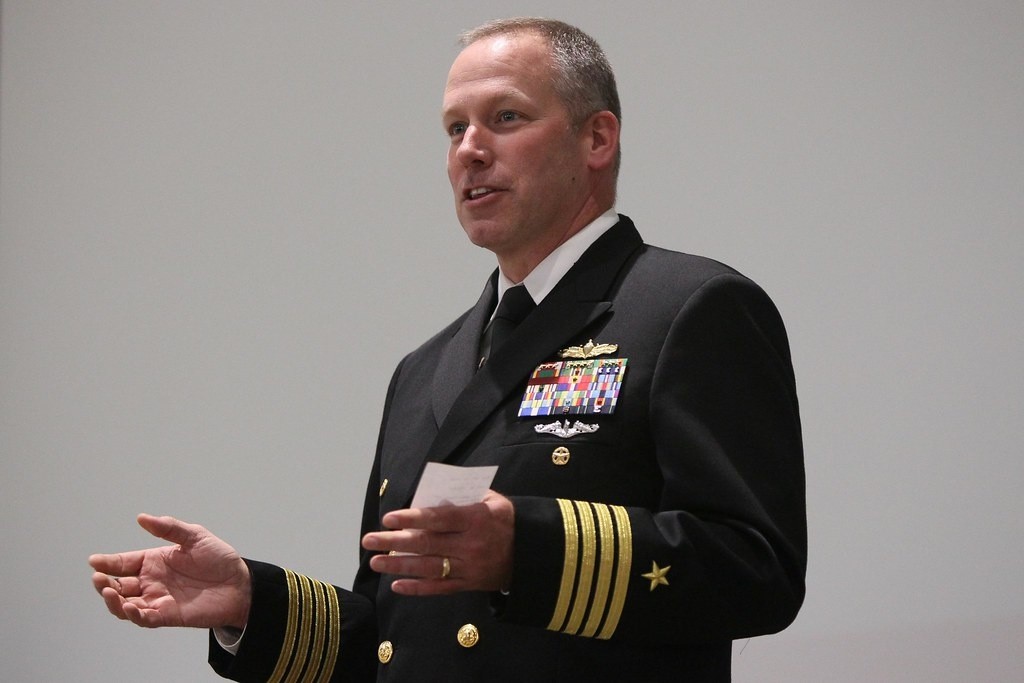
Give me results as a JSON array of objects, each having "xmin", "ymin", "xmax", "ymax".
[{"xmin": 89, "ymin": 15, "xmax": 808, "ymax": 683}]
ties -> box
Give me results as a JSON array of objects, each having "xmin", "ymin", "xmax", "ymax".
[{"xmin": 489, "ymin": 286, "xmax": 537, "ymax": 360}]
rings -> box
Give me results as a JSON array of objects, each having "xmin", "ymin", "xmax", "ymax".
[{"xmin": 439, "ymin": 556, "xmax": 452, "ymax": 581}]
